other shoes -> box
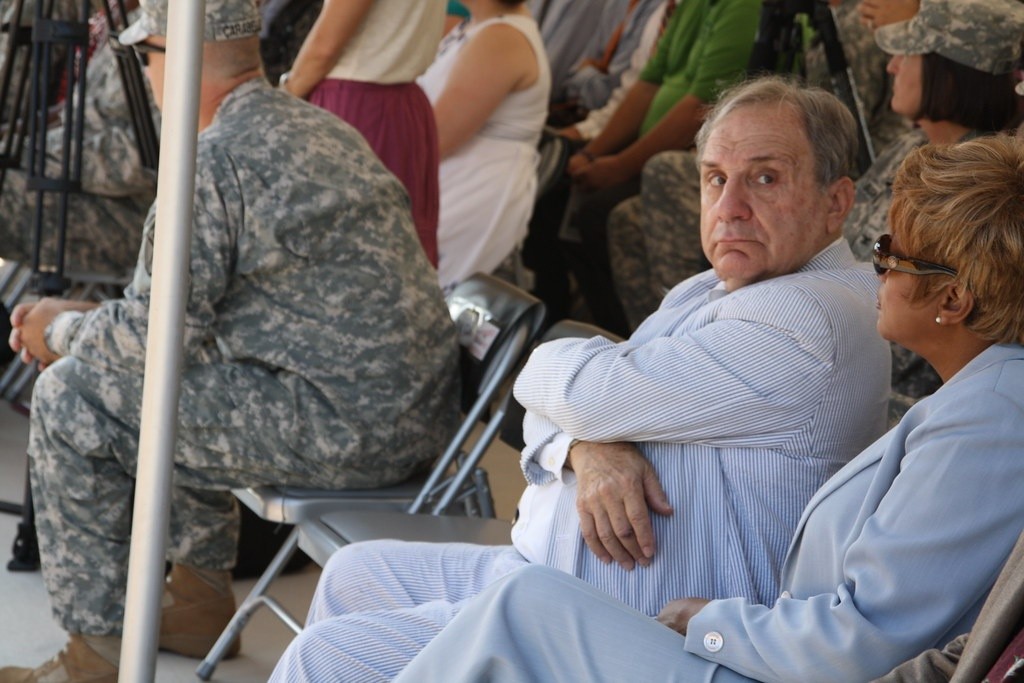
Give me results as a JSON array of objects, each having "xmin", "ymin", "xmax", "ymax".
[
  {"xmin": 0, "ymin": 632, "xmax": 121, "ymax": 683},
  {"xmin": 159, "ymin": 564, "xmax": 240, "ymax": 659}
]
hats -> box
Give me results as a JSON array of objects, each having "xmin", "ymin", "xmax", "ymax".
[{"xmin": 874, "ymin": 0, "xmax": 1024, "ymax": 76}]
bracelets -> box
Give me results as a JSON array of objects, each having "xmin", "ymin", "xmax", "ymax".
[
  {"xmin": 278, "ymin": 73, "xmax": 289, "ymax": 91},
  {"xmin": 580, "ymin": 150, "xmax": 595, "ymax": 162}
]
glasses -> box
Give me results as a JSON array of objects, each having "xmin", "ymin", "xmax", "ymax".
[
  {"xmin": 118, "ymin": 0, "xmax": 261, "ymax": 46},
  {"xmin": 872, "ymin": 234, "xmax": 957, "ymax": 277},
  {"xmin": 132, "ymin": 43, "xmax": 166, "ymax": 67}
]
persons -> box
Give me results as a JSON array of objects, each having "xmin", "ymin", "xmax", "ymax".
[
  {"xmin": 264, "ymin": 76, "xmax": 894, "ymax": 683},
  {"xmin": 3, "ymin": 1, "xmax": 551, "ymax": 683},
  {"xmin": 396, "ymin": 133, "xmax": 1024, "ymax": 683},
  {"xmin": 523, "ymin": 0, "xmax": 1024, "ymax": 430}
]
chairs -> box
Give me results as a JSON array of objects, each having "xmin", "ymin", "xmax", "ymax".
[{"xmin": 197, "ymin": 273, "xmax": 628, "ymax": 680}]
